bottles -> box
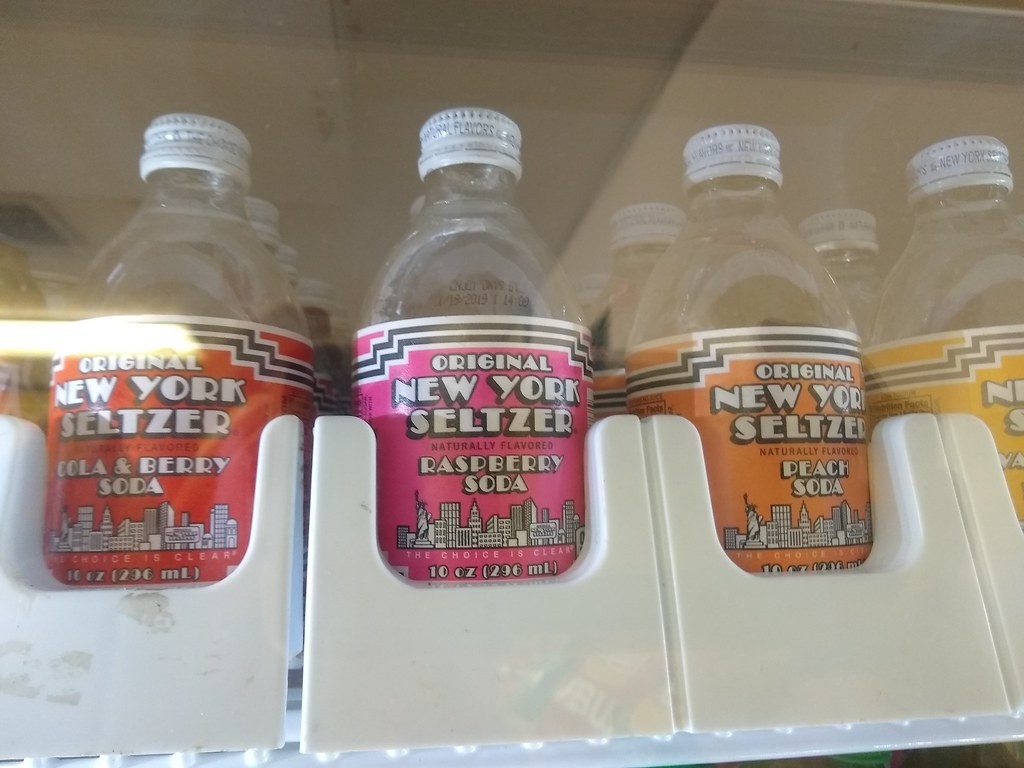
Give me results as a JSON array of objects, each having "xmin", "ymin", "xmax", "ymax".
[{"xmin": 0, "ymin": 106, "xmax": 1024, "ymax": 580}]
